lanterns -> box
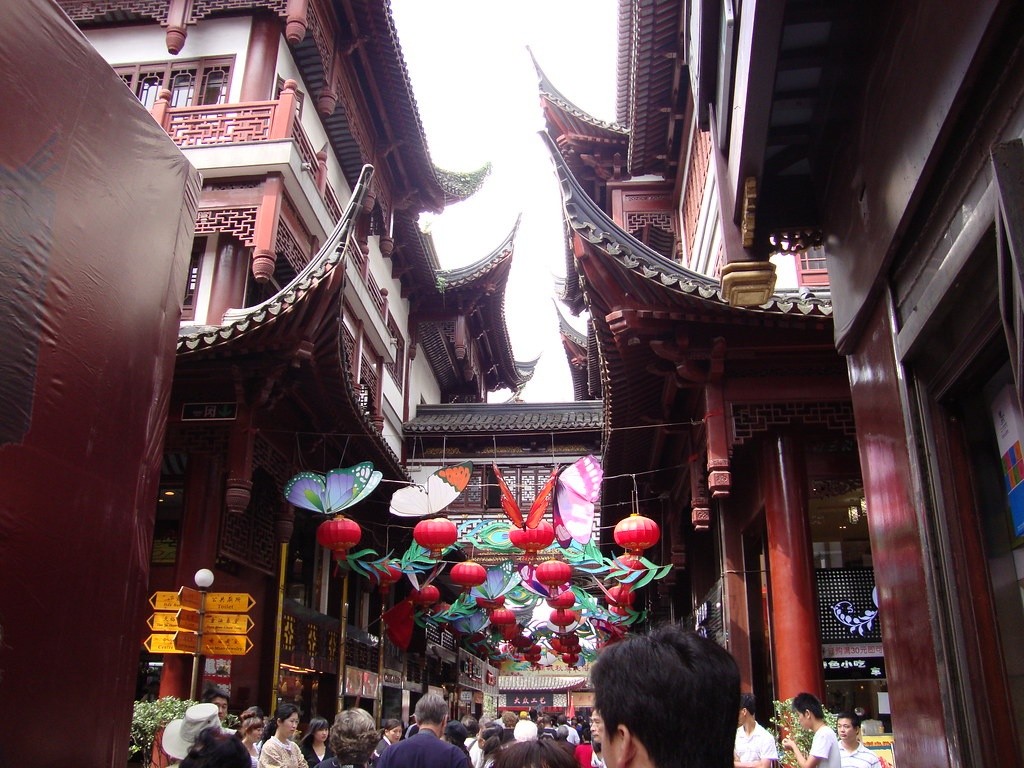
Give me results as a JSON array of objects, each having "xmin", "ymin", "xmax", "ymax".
[{"xmin": 316, "ymin": 511, "xmax": 661, "ymax": 668}]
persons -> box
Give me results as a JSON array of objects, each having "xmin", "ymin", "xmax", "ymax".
[
  {"xmin": 590, "ymin": 626, "xmax": 740, "ymax": 767},
  {"xmin": 162, "ymin": 690, "xmax": 884, "ymax": 768}
]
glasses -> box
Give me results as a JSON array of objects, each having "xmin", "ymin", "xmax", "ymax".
[{"xmin": 476, "ymin": 733, "xmax": 485, "ymax": 741}]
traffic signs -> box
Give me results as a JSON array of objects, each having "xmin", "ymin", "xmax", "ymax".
[{"xmin": 142, "ymin": 590, "xmax": 257, "ymax": 655}]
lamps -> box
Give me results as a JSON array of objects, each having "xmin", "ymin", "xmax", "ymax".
[
  {"xmin": 848, "ymin": 506, "xmax": 859, "ymax": 524},
  {"xmin": 860, "ymin": 498, "xmax": 868, "ymax": 515}
]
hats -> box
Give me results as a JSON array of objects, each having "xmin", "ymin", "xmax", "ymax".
[
  {"xmin": 514, "ymin": 720, "xmax": 538, "ymax": 742},
  {"xmin": 444, "ymin": 720, "xmax": 467, "ymax": 740},
  {"xmin": 520, "ymin": 711, "xmax": 527, "ymax": 718},
  {"xmin": 409, "ymin": 713, "xmax": 415, "ymax": 717},
  {"xmin": 162, "ymin": 703, "xmax": 237, "ymax": 759}
]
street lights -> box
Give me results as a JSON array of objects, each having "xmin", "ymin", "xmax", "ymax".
[{"xmin": 190, "ymin": 568, "xmax": 215, "ymax": 702}]
